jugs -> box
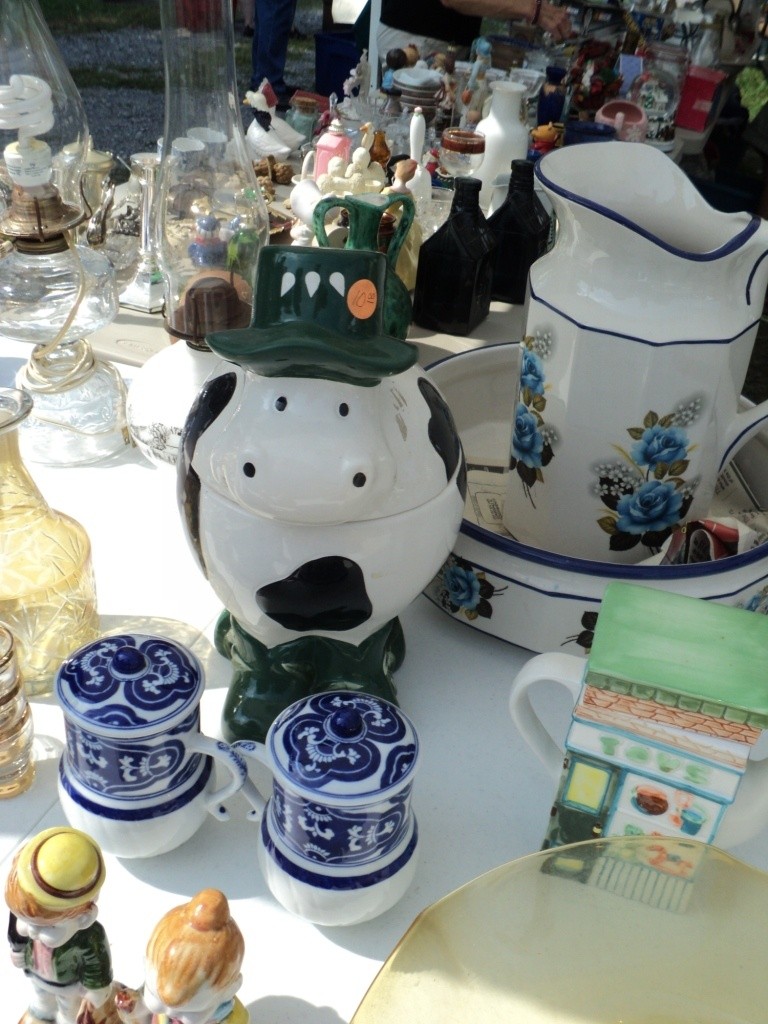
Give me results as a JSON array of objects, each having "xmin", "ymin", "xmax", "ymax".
[{"xmin": 500, "ymin": 141, "xmax": 768, "ymax": 567}]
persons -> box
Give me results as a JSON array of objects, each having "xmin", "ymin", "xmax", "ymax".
[
  {"xmin": 248, "ymin": 0, "xmax": 372, "ymax": 112},
  {"xmin": 114, "ymin": 887, "xmax": 249, "ymax": 1024},
  {"xmin": 289, "ymin": 146, "xmax": 424, "ymax": 291},
  {"xmin": 5, "ymin": 825, "xmax": 113, "ymax": 1024},
  {"xmin": 382, "ymin": 35, "xmax": 492, "ymax": 131},
  {"xmin": 377, "ymin": 0, "xmax": 572, "ymax": 62}
]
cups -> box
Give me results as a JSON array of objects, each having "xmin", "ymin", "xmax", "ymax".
[
  {"xmin": 228, "ymin": 688, "xmax": 420, "ymax": 928},
  {"xmin": 52, "ymin": 629, "xmax": 247, "ymax": 861},
  {"xmin": 155, "ymin": 124, "xmax": 227, "ymax": 169},
  {"xmin": 410, "ymin": 186, "xmax": 454, "ymax": 243},
  {"xmin": 506, "ymin": 651, "xmax": 768, "ymax": 848},
  {"xmin": 439, "ymin": 127, "xmax": 486, "ymax": 177}
]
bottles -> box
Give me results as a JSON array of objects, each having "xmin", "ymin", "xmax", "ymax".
[
  {"xmin": 144, "ymin": 0, "xmax": 272, "ymax": 340},
  {"xmin": 0, "ymin": 387, "xmax": 103, "ymax": 697},
  {"xmin": 0, "ymin": 623, "xmax": 37, "ymax": 801},
  {"xmin": 286, "ymin": 97, "xmax": 322, "ymax": 146},
  {"xmin": 470, "ymin": 80, "xmax": 529, "ymax": 220}
]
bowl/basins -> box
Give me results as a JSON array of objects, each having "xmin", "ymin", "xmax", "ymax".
[{"xmin": 421, "ymin": 342, "xmax": 768, "ymax": 660}]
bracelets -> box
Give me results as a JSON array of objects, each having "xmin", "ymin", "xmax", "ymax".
[{"xmin": 531, "ymin": 0, "xmax": 543, "ymax": 25}]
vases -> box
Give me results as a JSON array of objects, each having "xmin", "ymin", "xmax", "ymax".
[
  {"xmin": 470, "ymin": 81, "xmax": 527, "ymax": 216},
  {"xmin": 0, "ymin": 386, "xmax": 98, "ymax": 695}
]
